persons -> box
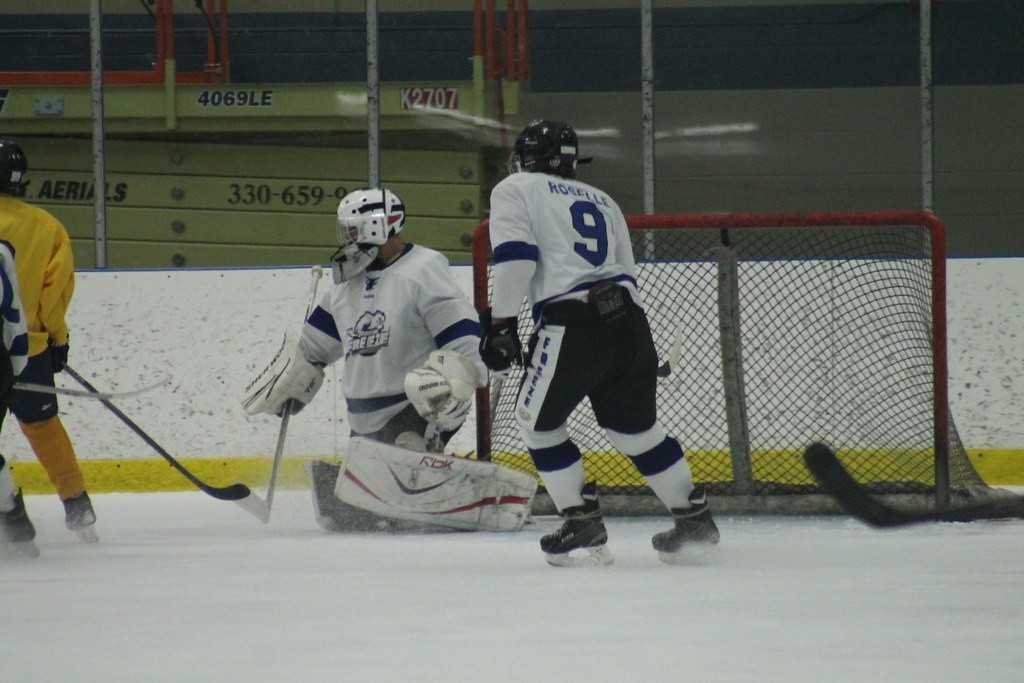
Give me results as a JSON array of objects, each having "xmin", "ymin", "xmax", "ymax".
[
  {"xmin": 0, "ymin": 138, "xmax": 102, "ymax": 564},
  {"xmin": 477, "ymin": 117, "xmax": 724, "ymax": 570},
  {"xmin": 237, "ymin": 186, "xmax": 538, "ymax": 533}
]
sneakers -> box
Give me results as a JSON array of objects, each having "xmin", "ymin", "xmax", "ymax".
[
  {"xmin": 652, "ymin": 483, "xmax": 726, "ymax": 566},
  {"xmin": 540, "ymin": 481, "xmax": 614, "ymax": 567},
  {"xmin": 0, "ymin": 487, "xmax": 39, "ymax": 557},
  {"xmin": 64, "ymin": 490, "xmax": 99, "ymax": 542}
]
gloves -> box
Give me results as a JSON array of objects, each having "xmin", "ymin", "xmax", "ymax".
[
  {"xmin": 478, "ymin": 307, "xmax": 521, "ymax": 371},
  {"xmin": 47, "ymin": 333, "xmax": 69, "ymax": 372},
  {"xmin": 14, "ymin": 371, "xmax": 36, "ymax": 402}
]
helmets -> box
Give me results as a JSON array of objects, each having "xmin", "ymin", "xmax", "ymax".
[
  {"xmin": 0, "ymin": 140, "xmax": 27, "ymax": 185},
  {"xmin": 508, "ymin": 118, "xmax": 578, "ymax": 179},
  {"xmin": 330, "ymin": 187, "xmax": 407, "ymax": 285}
]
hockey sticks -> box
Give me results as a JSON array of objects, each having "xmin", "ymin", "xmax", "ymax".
[
  {"xmin": 514, "ymin": 322, "xmax": 683, "ymax": 380},
  {"xmin": 13, "ymin": 373, "xmax": 178, "ymax": 403},
  {"xmin": 229, "ymin": 263, "xmax": 324, "ymax": 524},
  {"xmin": 63, "ymin": 363, "xmax": 252, "ymax": 502},
  {"xmin": 802, "ymin": 440, "xmax": 1023, "ymax": 533}
]
